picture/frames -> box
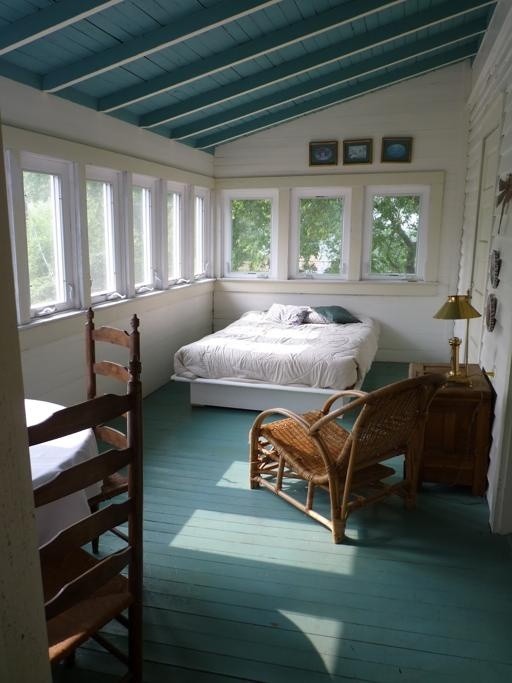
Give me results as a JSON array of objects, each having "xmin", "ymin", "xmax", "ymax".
[{"xmin": 308, "ymin": 136, "xmax": 413, "ymax": 166}]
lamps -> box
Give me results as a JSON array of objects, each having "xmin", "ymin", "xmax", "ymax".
[{"xmin": 432, "ymin": 293, "xmax": 482, "ymax": 385}]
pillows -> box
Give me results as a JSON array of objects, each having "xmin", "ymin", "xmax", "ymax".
[{"xmin": 262, "ymin": 303, "xmax": 362, "ymax": 327}]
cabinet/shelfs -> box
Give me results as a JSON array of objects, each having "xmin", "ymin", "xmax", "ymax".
[{"xmin": 402, "ymin": 363, "xmax": 498, "ymax": 505}]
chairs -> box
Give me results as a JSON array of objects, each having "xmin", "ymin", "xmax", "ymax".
[{"xmin": 247, "ymin": 372, "xmax": 447, "ymax": 545}]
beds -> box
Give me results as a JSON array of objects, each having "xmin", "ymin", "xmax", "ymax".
[{"xmin": 170, "ymin": 308, "xmax": 381, "ymax": 422}]
